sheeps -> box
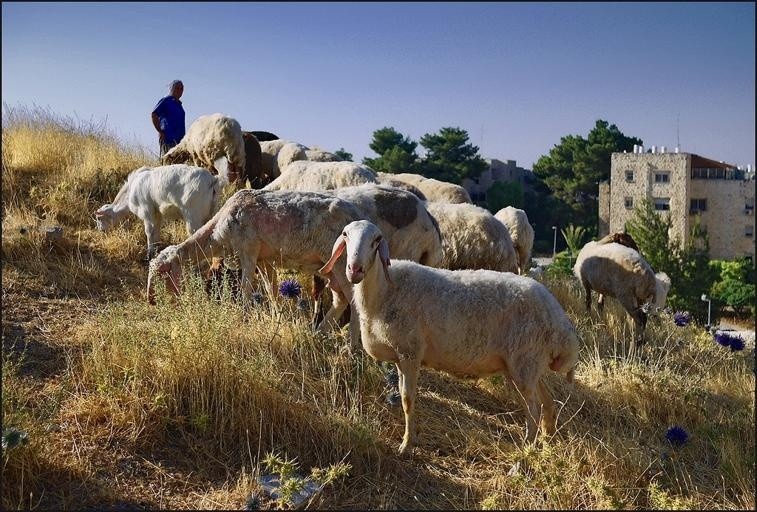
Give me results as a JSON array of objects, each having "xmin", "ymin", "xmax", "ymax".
[
  {"xmin": 146, "ymin": 185, "xmax": 442, "ymax": 347},
  {"xmin": 156, "ymin": 114, "xmax": 536, "ymax": 204},
  {"xmin": 492, "ymin": 206, "xmax": 535, "ymax": 275},
  {"xmin": 650, "ymin": 269, "xmax": 673, "ymax": 311},
  {"xmin": 316, "ymin": 219, "xmax": 580, "ymax": 458},
  {"xmin": 94, "ymin": 165, "xmax": 218, "ymax": 260},
  {"xmin": 423, "ymin": 201, "xmax": 518, "ymax": 274},
  {"xmin": 572, "ymin": 230, "xmax": 656, "ymax": 354}
]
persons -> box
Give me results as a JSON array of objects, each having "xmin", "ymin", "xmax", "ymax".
[{"xmin": 150, "ymin": 79, "xmax": 186, "ymax": 156}]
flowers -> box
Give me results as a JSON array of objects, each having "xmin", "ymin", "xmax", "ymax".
[
  {"xmin": 655, "ymin": 311, "xmax": 745, "ymax": 387},
  {"xmin": 270, "ymin": 278, "xmax": 302, "ymax": 345},
  {"xmin": 624, "ymin": 426, "xmax": 686, "ymax": 506}
]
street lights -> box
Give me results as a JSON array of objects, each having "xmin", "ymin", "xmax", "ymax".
[
  {"xmin": 552, "ymin": 226, "xmax": 557, "ymax": 256},
  {"xmin": 701, "ymin": 294, "xmax": 712, "ymax": 327}
]
